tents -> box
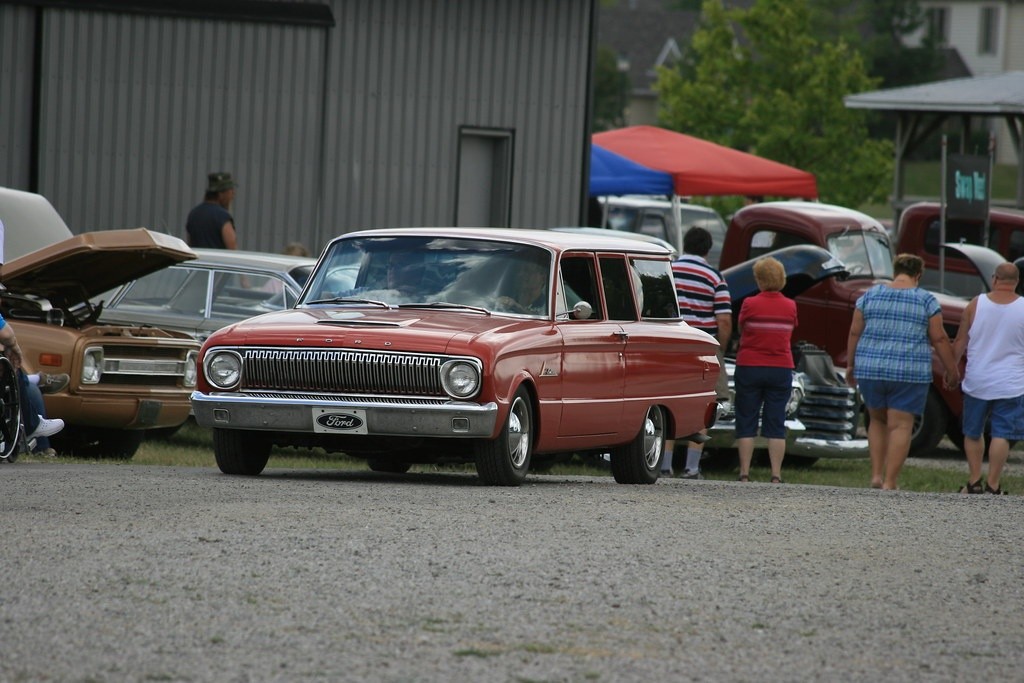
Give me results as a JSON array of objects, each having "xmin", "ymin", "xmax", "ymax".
[{"xmin": 589, "ymin": 125, "xmax": 820, "ymax": 258}]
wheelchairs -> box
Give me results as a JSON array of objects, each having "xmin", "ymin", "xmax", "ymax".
[{"xmin": 0, "ymin": 354, "xmax": 38, "ymax": 463}]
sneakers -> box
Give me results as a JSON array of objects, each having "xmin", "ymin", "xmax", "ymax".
[
  {"xmin": 32, "ymin": 446, "xmax": 56, "ymax": 457},
  {"xmin": 35, "ymin": 371, "xmax": 70, "ymax": 393},
  {"xmin": 27, "ymin": 414, "xmax": 64, "ymax": 440}
]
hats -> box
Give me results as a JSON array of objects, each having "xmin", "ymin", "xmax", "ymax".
[{"xmin": 206, "ymin": 171, "xmax": 239, "ymax": 192}]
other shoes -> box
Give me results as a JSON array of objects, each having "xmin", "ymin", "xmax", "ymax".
[
  {"xmin": 658, "ymin": 469, "xmax": 674, "ymax": 478},
  {"xmin": 770, "ymin": 477, "xmax": 782, "ymax": 483},
  {"xmin": 739, "ymin": 474, "xmax": 750, "ymax": 482},
  {"xmin": 679, "ymin": 470, "xmax": 703, "ymax": 480}
]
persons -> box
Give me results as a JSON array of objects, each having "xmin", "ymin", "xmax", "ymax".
[
  {"xmin": 184, "ymin": 171, "xmax": 311, "ymax": 294},
  {"xmin": 734, "ymin": 258, "xmax": 798, "ymax": 483},
  {"xmin": 659, "ymin": 227, "xmax": 732, "ymax": 480},
  {"xmin": 0, "ymin": 220, "xmax": 70, "ymax": 461},
  {"xmin": 846, "ymin": 254, "xmax": 960, "ymax": 491},
  {"xmin": 319, "ymin": 248, "xmax": 433, "ymax": 304},
  {"xmin": 426, "ymin": 253, "xmax": 549, "ymax": 317},
  {"xmin": 942, "ymin": 263, "xmax": 1024, "ymax": 495}
]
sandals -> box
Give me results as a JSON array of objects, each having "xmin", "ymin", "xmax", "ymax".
[
  {"xmin": 957, "ymin": 476, "xmax": 984, "ymax": 495},
  {"xmin": 983, "ymin": 482, "xmax": 1008, "ymax": 496}
]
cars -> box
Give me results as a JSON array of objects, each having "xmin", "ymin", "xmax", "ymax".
[
  {"xmin": 0, "ymin": 185, "xmax": 364, "ymax": 461},
  {"xmin": 189, "ymin": 226, "xmax": 722, "ymax": 486},
  {"xmin": 594, "ymin": 194, "xmax": 1024, "ymax": 471}
]
